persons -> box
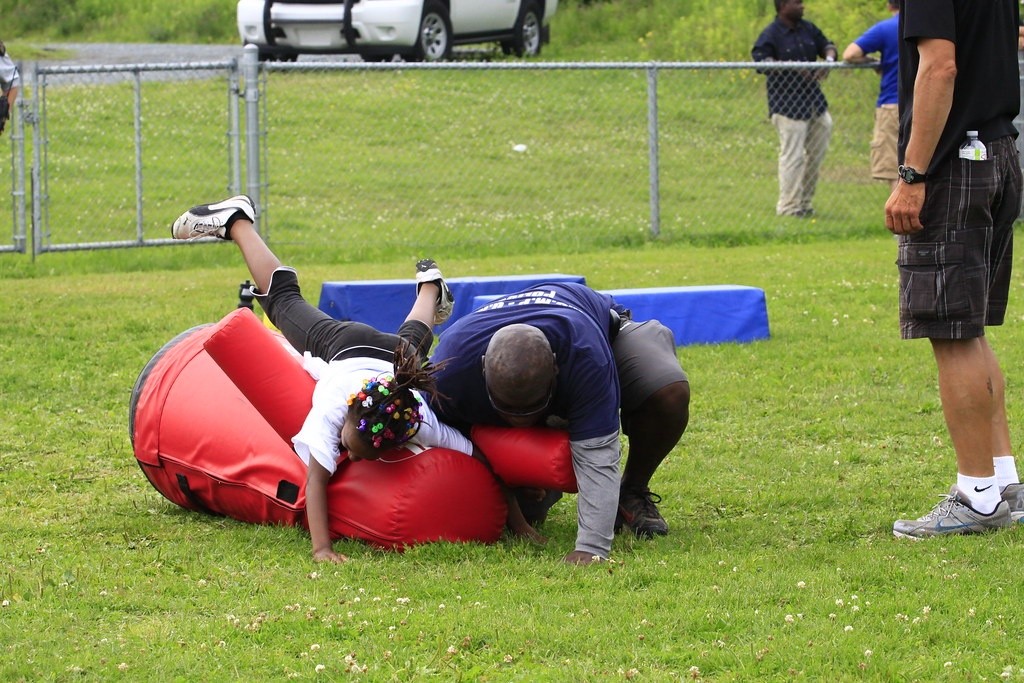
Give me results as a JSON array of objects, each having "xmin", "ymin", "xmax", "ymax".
[
  {"xmin": 171, "ymin": 193, "xmax": 549, "ymax": 566},
  {"xmin": 893, "ymin": 0, "xmax": 1024, "ymax": 541},
  {"xmin": 843, "ymin": 0, "xmax": 899, "ymax": 195},
  {"xmin": 752, "ymin": 0, "xmax": 838, "ymax": 219},
  {"xmin": 422, "ymin": 278, "xmax": 692, "ymax": 568}
]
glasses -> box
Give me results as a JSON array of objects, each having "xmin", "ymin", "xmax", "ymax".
[{"xmin": 483, "ymin": 381, "xmax": 553, "ymax": 417}]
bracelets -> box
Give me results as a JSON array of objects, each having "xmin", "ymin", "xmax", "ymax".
[{"xmin": 824, "ymin": 56, "xmax": 835, "ymax": 62}]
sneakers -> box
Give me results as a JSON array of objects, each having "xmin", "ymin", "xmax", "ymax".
[
  {"xmin": 615, "ymin": 481, "xmax": 669, "ymax": 539},
  {"xmin": 416, "ymin": 259, "xmax": 454, "ymax": 325},
  {"xmin": 1002, "ymin": 483, "xmax": 1024, "ymax": 521},
  {"xmin": 171, "ymin": 194, "xmax": 256, "ymax": 240},
  {"xmin": 893, "ymin": 485, "xmax": 1012, "ymax": 541}
]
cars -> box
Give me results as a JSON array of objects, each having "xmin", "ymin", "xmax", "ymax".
[{"xmin": 236, "ymin": 0, "xmax": 557, "ymax": 67}]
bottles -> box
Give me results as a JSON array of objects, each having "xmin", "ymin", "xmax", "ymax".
[{"xmin": 957, "ymin": 130, "xmax": 987, "ymax": 160}]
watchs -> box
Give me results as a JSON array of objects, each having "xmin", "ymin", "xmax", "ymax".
[{"xmin": 898, "ymin": 165, "xmax": 927, "ymax": 184}]
